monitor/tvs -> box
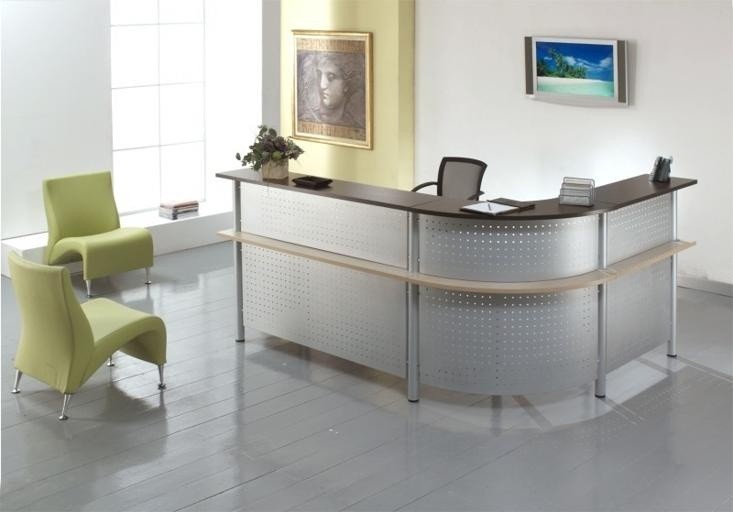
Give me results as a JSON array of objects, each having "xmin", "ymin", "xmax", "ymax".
[{"xmin": 525, "ymin": 36, "xmax": 628, "ymax": 107}]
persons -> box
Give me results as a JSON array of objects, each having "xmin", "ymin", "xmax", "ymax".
[{"xmin": 299, "ymin": 53, "xmax": 367, "ymax": 128}]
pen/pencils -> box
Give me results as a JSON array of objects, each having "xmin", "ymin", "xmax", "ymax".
[{"xmin": 488, "ymin": 203, "xmax": 491, "ymax": 211}]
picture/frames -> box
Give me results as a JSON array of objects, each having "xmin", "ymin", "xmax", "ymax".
[{"xmin": 290, "ymin": 31, "xmax": 374, "ymax": 149}]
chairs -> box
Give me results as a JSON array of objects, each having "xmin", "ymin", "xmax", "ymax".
[
  {"xmin": 42, "ymin": 170, "xmax": 154, "ymax": 299},
  {"xmin": 411, "ymin": 157, "xmax": 486, "ymax": 205},
  {"xmin": 7, "ymin": 253, "xmax": 167, "ymax": 422}
]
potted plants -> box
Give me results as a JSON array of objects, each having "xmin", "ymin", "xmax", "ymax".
[{"xmin": 233, "ymin": 123, "xmax": 304, "ymax": 182}]
